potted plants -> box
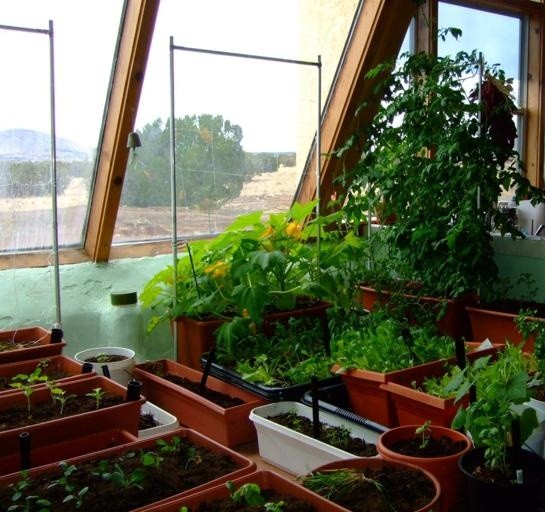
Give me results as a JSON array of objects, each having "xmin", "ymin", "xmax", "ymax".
[
  {"xmin": 329, "ymin": 305, "xmax": 508, "ymax": 427},
  {"xmin": 137, "ymin": 393, "xmax": 179, "ymax": 439},
  {"xmin": 136, "ymin": 200, "xmax": 375, "ymax": 448},
  {"xmin": 2, "ymin": 427, "xmax": 255, "ymax": 511},
  {"xmin": 0, "ymin": 325, "xmax": 68, "ymax": 356},
  {"xmin": 300, "ymin": 456, "xmax": 442, "ymax": 510},
  {"xmin": 149, "ymin": 469, "xmax": 348, "ymax": 511},
  {"xmin": 199, "ymin": 311, "xmax": 453, "ymax": 402},
  {"xmin": 248, "ymin": 353, "xmax": 379, "ymax": 483},
  {"xmin": 378, "ymin": 341, "xmax": 542, "ymax": 430},
  {"xmin": 77, "ymin": 347, "xmax": 138, "ymax": 387},
  {"xmin": 2, "ymin": 375, "xmax": 146, "ymax": 477},
  {"xmin": 378, "ymin": 418, "xmax": 472, "ymax": 509},
  {"xmin": 376, "ymin": 27, "xmax": 542, "ymax": 429},
  {"xmin": 509, "ymin": 303, "xmax": 543, "ymax": 456},
  {"xmin": 3, "ymin": 354, "xmax": 96, "ymax": 393},
  {"xmin": 457, "ymin": 340, "xmax": 543, "ymax": 512}
]
